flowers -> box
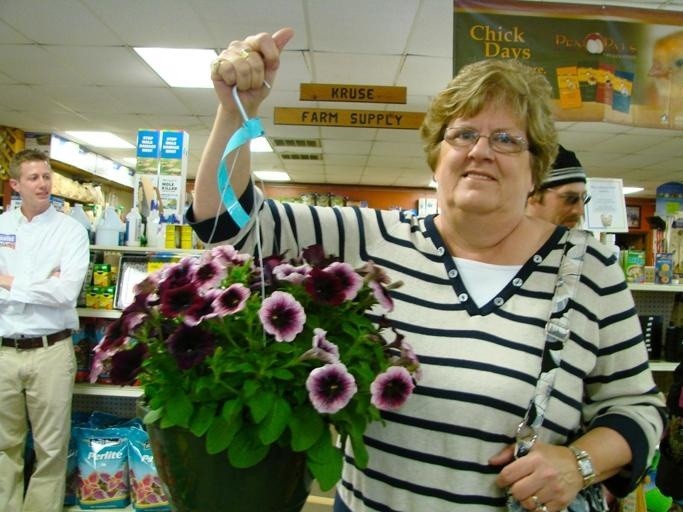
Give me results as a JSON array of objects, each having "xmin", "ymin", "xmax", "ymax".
[{"xmin": 91, "ymin": 245, "xmax": 423, "ymax": 491}]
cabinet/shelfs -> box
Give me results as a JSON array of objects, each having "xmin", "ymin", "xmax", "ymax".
[
  {"xmin": 627, "ymin": 273, "xmax": 682, "ymax": 372},
  {"xmin": 61, "ymin": 244, "xmax": 208, "ymax": 512},
  {"xmin": 24, "ymin": 132, "xmax": 139, "ymax": 245}
]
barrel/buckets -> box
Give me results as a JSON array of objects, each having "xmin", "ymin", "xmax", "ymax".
[
  {"xmin": 96, "ymin": 204, "xmax": 120, "ymax": 245},
  {"xmin": 68, "ymin": 203, "xmax": 91, "ymax": 235}
]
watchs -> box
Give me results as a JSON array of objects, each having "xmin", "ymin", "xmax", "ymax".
[{"xmin": 569, "ymin": 443, "xmax": 595, "ymax": 488}]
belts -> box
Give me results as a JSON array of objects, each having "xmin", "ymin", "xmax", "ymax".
[{"xmin": 1, "ymin": 329, "xmax": 70, "ymax": 352}]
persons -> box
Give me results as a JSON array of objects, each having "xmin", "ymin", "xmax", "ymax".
[
  {"xmin": 523, "ymin": 142, "xmax": 592, "ymax": 230},
  {"xmin": 0, "ymin": 148, "xmax": 89, "ymax": 511},
  {"xmin": 182, "ymin": 24, "xmax": 671, "ymax": 512}
]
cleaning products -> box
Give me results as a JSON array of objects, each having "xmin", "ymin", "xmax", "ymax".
[{"xmin": 126, "ymin": 207, "xmax": 142, "ymax": 245}]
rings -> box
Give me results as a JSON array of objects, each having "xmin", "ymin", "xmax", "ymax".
[
  {"xmin": 528, "ymin": 494, "xmax": 539, "ymax": 502},
  {"xmin": 212, "ymin": 58, "xmax": 227, "ymax": 77},
  {"xmin": 539, "ymin": 504, "xmax": 547, "ymax": 511},
  {"xmin": 238, "ymin": 47, "xmax": 254, "ymax": 60}
]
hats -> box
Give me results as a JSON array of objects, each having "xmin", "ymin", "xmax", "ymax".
[{"xmin": 540, "ymin": 144, "xmax": 587, "ymax": 190}]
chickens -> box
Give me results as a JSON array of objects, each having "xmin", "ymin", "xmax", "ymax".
[{"xmin": 647, "ymin": 31, "xmax": 682, "ymax": 109}]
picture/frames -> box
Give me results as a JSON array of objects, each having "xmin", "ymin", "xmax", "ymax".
[
  {"xmin": 626, "ymin": 205, "xmax": 642, "ymax": 229},
  {"xmin": 585, "ymin": 177, "xmax": 629, "ymax": 234}
]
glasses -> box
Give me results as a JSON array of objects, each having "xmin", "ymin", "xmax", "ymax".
[
  {"xmin": 442, "ymin": 126, "xmax": 531, "ymax": 155},
  {"xmin": 544, "ymin": 187, "xmax": 592, "ymax": 204}
]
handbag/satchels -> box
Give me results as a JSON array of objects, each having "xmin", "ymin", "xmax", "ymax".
[{"xmin": 505, "ymin": 446, "xmax": 611, "ymax": 512}]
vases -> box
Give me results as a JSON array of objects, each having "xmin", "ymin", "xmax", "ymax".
[{"xmin": 135, "ymin": 393, "xmax": 313, "ymax": 512}]
